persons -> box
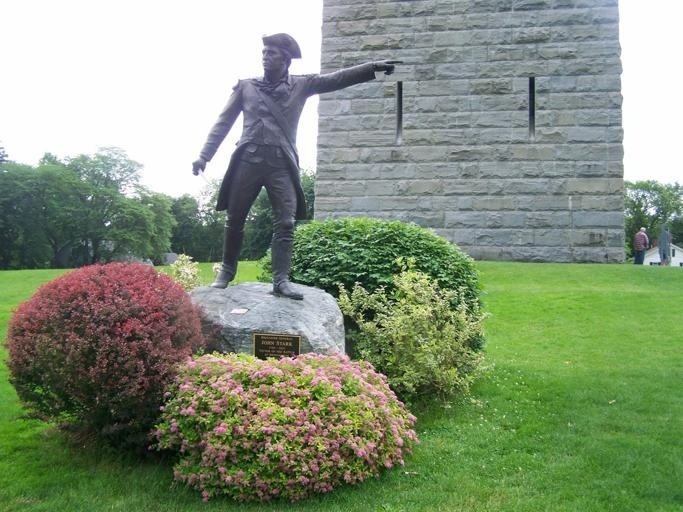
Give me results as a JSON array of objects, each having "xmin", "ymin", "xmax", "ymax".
[
  {"xmin": 191, "ymin": 33, "xmax": 404, "ymax": 301},
  {"xmin": 657, "ymin": 225, "xmax": 673, "ymax": 267},
  {"xmin": 632, "ymin": 226, "xmax": 649, "ymax": 264}
]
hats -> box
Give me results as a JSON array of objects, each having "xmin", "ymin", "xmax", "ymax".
[{"xmin": 264, "ymin": 32, "xmax": 300, "ymax": 60}]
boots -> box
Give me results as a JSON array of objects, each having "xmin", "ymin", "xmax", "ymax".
[{"xmin": 214, "ymin": 235, "xmax": 302, "ymax": 298}]
